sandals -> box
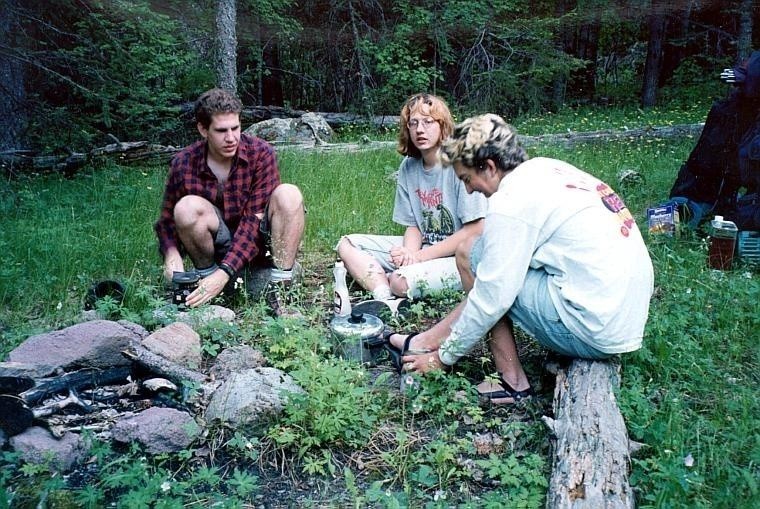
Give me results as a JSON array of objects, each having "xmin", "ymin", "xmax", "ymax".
[
  {"xmin": 469, "ymin": 374, "xmax": 536, "ymax": 405},
  {"xmin": 381, "ymin": 329, "xmax": 422, "ymax": 375}
]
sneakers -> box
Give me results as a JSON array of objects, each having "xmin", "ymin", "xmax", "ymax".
[
  {"xmin": 382, "ymin": 295, "xmax": 410, "ymax": 316},
  {"xmin": 263, "ymin": 277, "xmax": 303, "ymax": 318},
  {"xmin": 212, "ymin": 271, "xmax": 237, "ymax": 308}
]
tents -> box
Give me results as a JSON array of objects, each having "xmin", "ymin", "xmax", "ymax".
[{"xmin": 667, "ymin": 46, "xmax": 760, "ymax": 245}]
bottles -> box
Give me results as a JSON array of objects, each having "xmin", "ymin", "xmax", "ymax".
[{"xmin": 333, "ymin": 258, "xmax": 352, "ymax": 315}]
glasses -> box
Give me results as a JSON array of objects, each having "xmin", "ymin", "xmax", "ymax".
[{"xmin": 405, "ymin": 115, "xmax": 435, "ymax": 132}]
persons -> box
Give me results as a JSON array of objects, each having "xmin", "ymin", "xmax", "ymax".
[
  {"xmin": 332, "ymin": 92, "xmax": 489, "ymax": 317},
  {"xmin": 152, "ymin": 86, "xmax": 307, "ymax": 318},
  {"xmin": 379, "ymin": 111, "xmax": 656, "ymax": 405}
]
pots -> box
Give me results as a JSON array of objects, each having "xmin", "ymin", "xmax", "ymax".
[{"xmin": 330, "ymin": 312, "xmax": 401, "ymax": 369}]
